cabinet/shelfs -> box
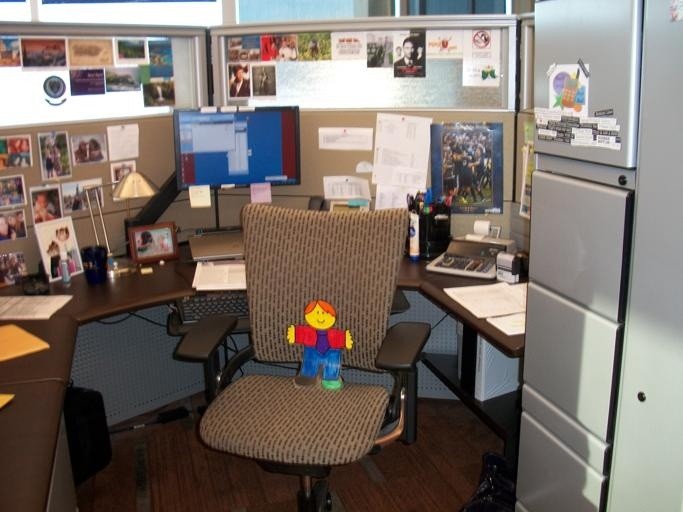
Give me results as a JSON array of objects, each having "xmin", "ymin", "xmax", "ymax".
[{"xmin": 514, "ymin": 0, "xmax": 648, "ymax": 512}]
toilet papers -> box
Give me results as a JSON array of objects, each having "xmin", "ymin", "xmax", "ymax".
[{"xmin": 464, "ymin": 220, "xmax": 491, "ymax": 241}]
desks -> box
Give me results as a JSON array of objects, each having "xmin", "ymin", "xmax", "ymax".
[{"xmin": 0, "ymin": 243, "xmax": 527, "ymax": 512}]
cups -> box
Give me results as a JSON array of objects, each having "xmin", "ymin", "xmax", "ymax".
[{"xmin": 80, "ymin": 246, "xmax": 107, "ymax": 287}]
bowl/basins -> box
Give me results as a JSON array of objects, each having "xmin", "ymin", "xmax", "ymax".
[{"xmin": 20, "ymin": 273, "xmax": 50, "ymax": 296}]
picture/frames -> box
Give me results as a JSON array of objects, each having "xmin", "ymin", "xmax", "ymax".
[
  {"xmin": 0, "ymin": 209, "xmax": 28, "ymax": 242},
  {"xmin": 0, "ymin": 174, "xmax": 28, "ymax": 209},
  {"xmin": 127, "ymin": 223, "xmax": 180, "ymax": 262},
  {"xmin": 33, "ymin": 217, "xmax": 84, "ymax": 283},
  {"xmin": 30, "ymin": 183, "xmax": 63, "ymax": 224},
  {"xmin": 110, "ymin": 160, "xmax": 137, "ymax": 203},
  {"xmin": 37, "ymin": 131, "xmax": 72, "ymax": 180}
]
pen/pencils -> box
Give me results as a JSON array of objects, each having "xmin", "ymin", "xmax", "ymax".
[{"xmin": 407, "ymin": 188, "xmax": 452, "ymax": 216}]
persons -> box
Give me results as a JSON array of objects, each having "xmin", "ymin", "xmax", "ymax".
[
  {"xmin": 0, "ymin": 134, "xmax": 169, "ymax": 286},
  {"xmin": 262, "ymin": 35, "xmax": 296, "ymax": 60},
  {"xmin": 306, "ymin": 38, "xmax": 321, "ymax": 60},
  {"xmin": 394, "ymin": 35, "xmax": 425, "ymax": 76},
  {"xmin": 442, "ymin": 126, "xmax": 492, "ymax": 204},
  {"xmin": 259, "ymin": 72, "xmax": 268, "ymax": 94},
  {"xmin": 230, "ymin": 64, "xmax": 250, "ymax": 96}
]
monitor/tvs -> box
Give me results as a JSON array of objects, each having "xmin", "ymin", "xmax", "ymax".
[{"xmin": 124, "ymin": 106, "xmax": 301, "ymax": 258}]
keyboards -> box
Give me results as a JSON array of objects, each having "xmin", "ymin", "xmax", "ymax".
[{"xmin": 176, "ymin": 293, "xmax": 249, "ymax": 324}]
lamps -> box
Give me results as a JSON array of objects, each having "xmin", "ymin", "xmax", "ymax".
[{"xmin": 83, "ymin": 170, "xmax": 161, "ymax": 268}]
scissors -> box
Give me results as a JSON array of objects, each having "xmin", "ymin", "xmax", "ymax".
[{"xmin": 424, "ymin": 188, "xmax": 432, "ymax": 205}]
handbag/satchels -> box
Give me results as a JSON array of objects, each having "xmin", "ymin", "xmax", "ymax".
[{"xmin": 458, "ymin": 452, "xmax": 516, "ymax": 512}]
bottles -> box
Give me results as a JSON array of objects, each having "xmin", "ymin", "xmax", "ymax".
[{"xmin": 58, "ymin": 250, "xmax": 71, "ymax": 284}]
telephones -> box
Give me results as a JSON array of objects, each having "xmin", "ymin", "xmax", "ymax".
[{"xmin": 308, "ymin": 195, "xmax": 370, "ymax": 213}]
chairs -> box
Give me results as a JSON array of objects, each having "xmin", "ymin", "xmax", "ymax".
[{"xmin": 172, "ymin": 204, "xmax": 432, "ymax": 512}]
